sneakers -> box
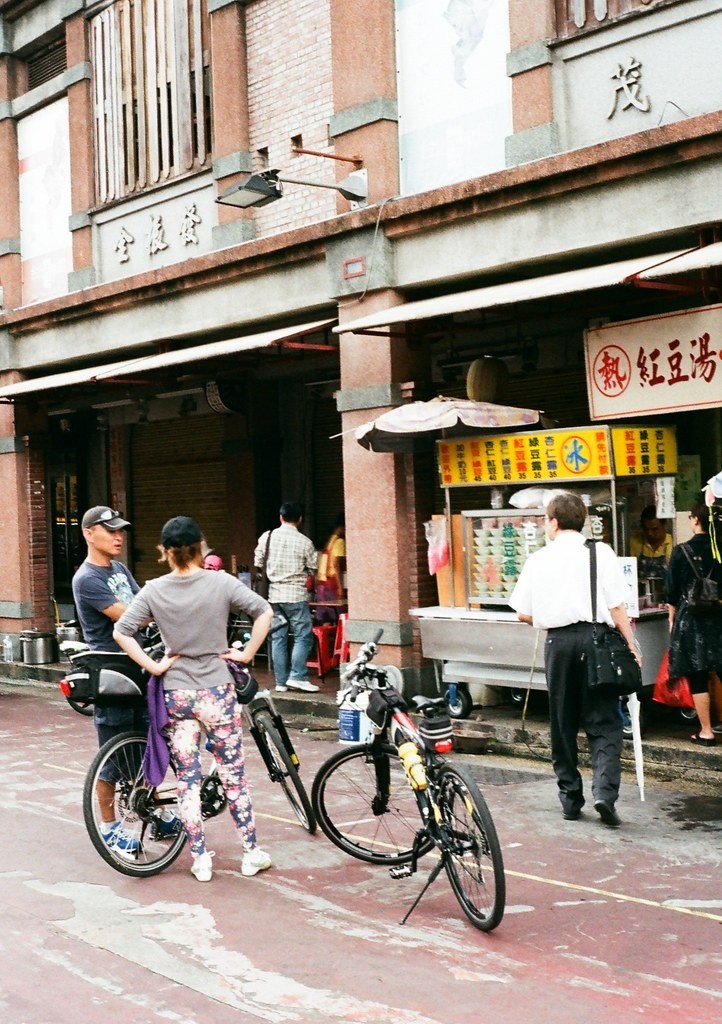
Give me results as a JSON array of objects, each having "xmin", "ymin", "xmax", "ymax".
[
  {"xmin": 241, "ymin": 849, "xmax": 270, "ymax": 877},
  {"xmin": 190, "ymin": 851, "xmax": 216, "ymax": 882},
  {"xmin": 275, "ymin": 685, "xmax": 288, "ymax": 692},
  {"xmin": 149, "ymin": 810, "xmax": 181, "ymax": 840},
  {"xmin": 99, "ymin": 821, "xmax": 140, "ymax": 853},
  {"xmin": 286, "ymin": 680, "xmax": 319, "ymax": 692}
]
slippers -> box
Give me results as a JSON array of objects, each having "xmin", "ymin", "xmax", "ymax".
[{"xmin": 691, "ymin": 732, "xmax": 715, "ymax": 746}]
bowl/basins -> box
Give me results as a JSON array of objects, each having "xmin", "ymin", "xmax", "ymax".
[
  {"xmin": 453, "ymin": 730, "xmax": 489, "ymax": 754},
  {"xmin": 56, "ymin": 627, "xmax": 83, "ymax": 642},
  {"xmin": 474, "ymin": 528, "xmax": 546, "ymax": 598}
]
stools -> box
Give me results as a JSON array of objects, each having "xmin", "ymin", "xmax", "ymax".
[
  {"xmin": 332, "ymin": 612, "xmax": 350, "ymax": 665},
  {"xmin": 306, "ymin": 625, "xmax": 337, "ymax": 676}
]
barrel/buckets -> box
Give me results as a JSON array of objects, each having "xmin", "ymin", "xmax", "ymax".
[{"xmin": 335, "ymin": 689, "xmax": 375, "ymax": 745}]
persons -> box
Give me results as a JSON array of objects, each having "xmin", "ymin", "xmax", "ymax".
[
  {"xmin": 199, "ymin": 549, "xmax": 228, "ymax": 572},
  {"xmin": 113, "ymin": 517, "xmax": 273, "ymax": 881},
  {"xmin": 666, "ymin": 501, "xmax": 722, "ymax": 747},
  {"xmin": 507, "ymin": 494, "xmax": 642, "ymax": 826},
  {"xmin": 327, "ymin": 531, "xmax": 346, "ymax": 626},
  {"xmin": 72, "ymin": 507, "xmax": 188, "ymax": 853},
  {"xmin": 630, "ymin": 504, "xmax": 673, "ymax": 566},
  {"xmin": 254, "ymin": 502, "xmax": 319, "ymax": 691}
]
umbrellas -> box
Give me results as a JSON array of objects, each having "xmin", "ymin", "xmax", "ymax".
[{"xmin": 354, "ymin": 396, "xmax": 560, "ymax": 609}]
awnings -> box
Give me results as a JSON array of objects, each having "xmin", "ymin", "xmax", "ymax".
[
  {"xmin": 332, "ymin": 242, "xmax": 722, "ymax": 332},
  {"xmin": 0, "ymin": 317, "xmax": 339, "ymax": 397}
]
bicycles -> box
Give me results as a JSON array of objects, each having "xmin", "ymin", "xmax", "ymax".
[
  {"xmin": 310, "ymin": 627, "xmax": 506, "ymax": 931},
  {"xmin": 81, "ymin": 621, "xmax": 316, "ymax": 877}
]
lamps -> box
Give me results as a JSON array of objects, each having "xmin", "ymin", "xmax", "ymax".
[{"xmin": 214, "ymin": 168, "xmax": 367, "ymax": 209}]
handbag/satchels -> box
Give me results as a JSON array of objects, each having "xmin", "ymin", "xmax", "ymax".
[
  {"xmin": 652, "ymin": 647, "xmax": 696, "ymax": 708},
  {"xmin": 255, "ymin": 529, "xmax": 272, "ymax": 603},
  {"xmin": 581, "ymin": 624, "xmax": 641, "ymax": 695}
]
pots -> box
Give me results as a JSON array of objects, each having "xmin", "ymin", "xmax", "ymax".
[{"xmin": 19, "ymin": 628, "xmax": 58, "ymax": 664}]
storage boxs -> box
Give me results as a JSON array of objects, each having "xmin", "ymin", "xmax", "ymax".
[{"xmin": 460, "ymin": 508, "xmax": 550, "ymax": 611}]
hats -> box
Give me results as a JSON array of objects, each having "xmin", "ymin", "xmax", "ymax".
[
  {"xmin": 81, "ymin": 506, "xmax": 132, "ymax": 531},
  {"xmin": 161, "ymin": 516, "xmax": 201, "ymax": 546}
]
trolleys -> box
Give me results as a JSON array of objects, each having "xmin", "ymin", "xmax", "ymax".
[{"xmin": 407, "ymin": 422, "xmax": 701, "ymax": 736}]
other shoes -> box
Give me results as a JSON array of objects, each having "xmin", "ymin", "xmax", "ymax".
[
  {"xmin": 561, "ymin": 807, "xmax": 580, "ymax": 820},
  {"xmin": 592, "ymin": 799, "xmax": 620, "ymax": 824}
]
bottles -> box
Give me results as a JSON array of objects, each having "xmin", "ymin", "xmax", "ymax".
[
  {"xmin": 398, "ymin": 738, "xmax": 427, "ymax": 789},
  {"xmin": 3, "ymin": 636, "xmax": 13, "ymax": 662}
]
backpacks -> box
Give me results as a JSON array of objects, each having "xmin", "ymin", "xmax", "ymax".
[{"xmin": 675, "ymin": 542, "xmax": 720, "ymax": 618}]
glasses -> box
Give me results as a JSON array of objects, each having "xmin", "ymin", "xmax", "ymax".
[
  {"xmin": 92, "ymin": 510, "xmax": 124, "ymax": 524},
  {"xmin": 688, "ymin": 515, "xmax": 693, "ymax": 520}
]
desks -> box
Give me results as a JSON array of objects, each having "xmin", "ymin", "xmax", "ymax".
[
  {"xmin": 229, "ymin": 609, "xmax": 274, "ymax": 671},
  {"xmin": 307, "ymin": 599, "xmax": 348, "ymax": 623}
]
